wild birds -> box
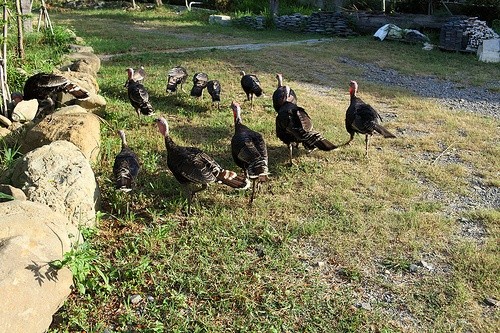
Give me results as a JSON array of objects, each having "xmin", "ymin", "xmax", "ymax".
[
  {"xmin": 191, "ymin": 69, "xmax": 207, "ymax": 105},
  {"xmin": 153, "ymin": 116, "xmax": 250, "ymax": 215},
  {"xmin": 274, "ymin": 72, "xmax": 296, "ymax": 113},
  {"xmin": 206, "ymin": 80, "xmax": 224, "ymax": 113},
  {"xmin": 126, "ymin": 68, "xmax": 155, "ymax": 124},
  {"xmin": 343, "ymin": 79, "xmax": 397, "ymax": 159},
  {"xmin": 166, "ymin": 64, "xmax": 187, "ymax": 101},
  {"xmin": 125, "ymin": 65, "xmax": 144, "ymax": 88},
  {"xmin": 277, "ymin": 84, "xmax": 337, "ymax": 168},
  {"xmin": 114, "ymin": 130, "xmax": 142, "ymax": 197},
  {"xmin": 228, "ymin": 101, "xmax": 271, "ymax": 205},
  {"xmin": 11, "ymin": 72, "xmax": 89, "ymax": 120},
  {"xmin": 238, "ymin": 71, "xmax": 265, "ymax": 112}
]
